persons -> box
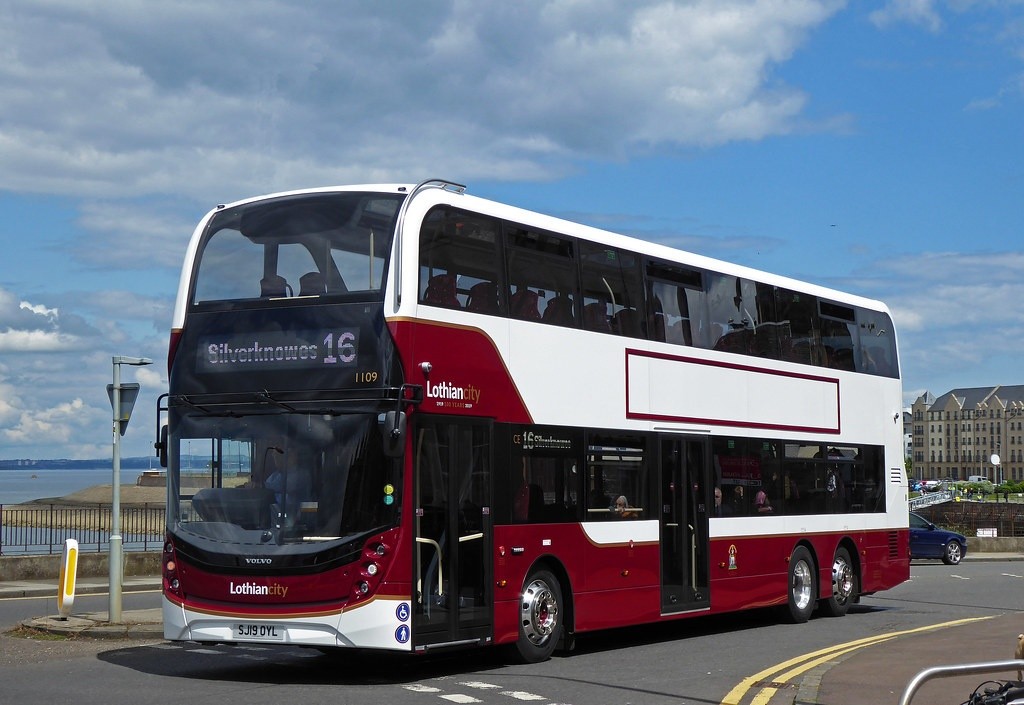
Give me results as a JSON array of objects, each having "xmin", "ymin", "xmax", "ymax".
[
  {"xmin": 713, "ymin": 487, "xmax": 733, "ymax": 517},
  {"xmin": 608, "ymin": 495, "xmax": 638, "ymax": 520},
  {"xmin": 920, "ymin": 489, "xmax": 928, "ymax": 498},
  {"xmin": 753, "ymin": 491, "xmax": 775, "ymax": 514},
  {"xmin": 940, "ymin": 485, "xmax": 953, "ymax": 493},
  {"xmin": 829, "ymin": 448, "xmax": 844, "ymax": 456},
  {"xmin": 969, "ymin": 488, "xmax": 973, "ymax": 497},
  {"xmin": 246, "ymin": 450, "xmax": 287, "ymax": 503}
]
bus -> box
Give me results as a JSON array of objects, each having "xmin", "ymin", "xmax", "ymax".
[{"xmin": 151, "ymin": 178, "xmax": 911, "ymax": 659}]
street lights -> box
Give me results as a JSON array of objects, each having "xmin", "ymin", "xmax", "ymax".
[
  {"xmin": 995, "ymin": 442, "xmax": 1002, "ymax": 484},
  {"xmin": 109, "ymin": 355, "xmax": 154, "ymax": 626}
]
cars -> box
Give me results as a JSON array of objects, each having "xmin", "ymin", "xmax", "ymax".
[
  {"xmin": 908, "ymin": 479, "xmax": 941, "ymax": 491},
  {"xmin": 909, "ymin": 512, "xmax": 969, "ymax": 565}
]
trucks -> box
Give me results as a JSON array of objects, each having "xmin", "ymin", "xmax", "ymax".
[{"xmin": 968, "ymin": 475, "xmax": 988, "ymax": 482}]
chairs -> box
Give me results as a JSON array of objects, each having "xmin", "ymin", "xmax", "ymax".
[
  {"xmin": 528, "ymin": 458, "xmax": 865, "ymax": 525},
  {"xmin": 258, "ymin": 272, "xmax": 886, "ymax": 377}
]
